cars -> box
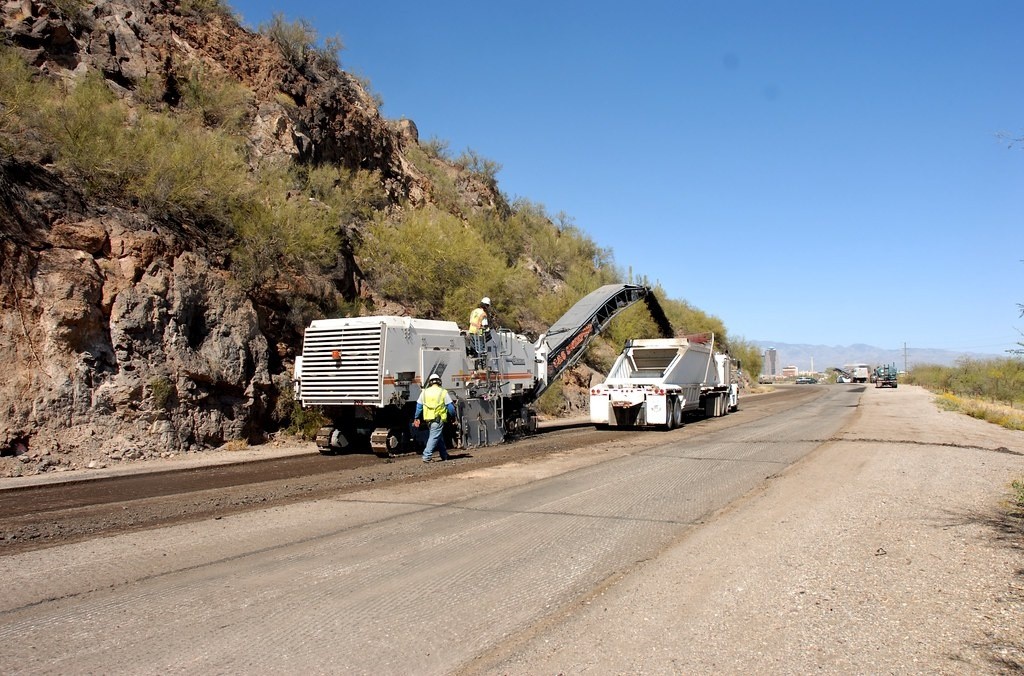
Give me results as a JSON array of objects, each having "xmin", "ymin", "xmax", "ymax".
[
  {"xmin": 870, "ymin": 374, "xmax": 876, "ymax": 382},
  {"xmin": 796, "ymin": 377, "xmax": 815, "ymax": 384}
]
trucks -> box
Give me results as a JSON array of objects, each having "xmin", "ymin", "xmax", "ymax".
[
  {"xmin": 852, "ymin": 368, "xmax": 868, "ymax": 383},
  {"xmin": 759, "ymin": 375, "xmax": 772, "ymax": 384}
]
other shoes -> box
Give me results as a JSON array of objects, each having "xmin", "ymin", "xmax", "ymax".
[
  {"xmin": 424, "ymin": 459, "xmax": 435, "ymax": 463},
  {"xmin": 442, "ymin": 454, "xmax": 449, "ymax": 461}
]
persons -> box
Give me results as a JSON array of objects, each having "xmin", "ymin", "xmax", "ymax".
[
  {"xmin": 414, "ymin": 373, "xmax": 455, "ymax": 463},
  {"xmin": 469, "ymin": 296, "xmax": 492, "ymax": 371}
]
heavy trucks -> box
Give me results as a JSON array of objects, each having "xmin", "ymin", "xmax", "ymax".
[{"xmin": 589, "ymin": 331, "xmax": 743, "ymax": 431}]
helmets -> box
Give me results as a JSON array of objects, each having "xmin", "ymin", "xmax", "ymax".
[
  {"xmin": 481, "ymin": 297, "xmax": 490, "ymax": 306},
  {"xmin": 429, "ymin": 373, "xmax": 440, "ymax": 381}
]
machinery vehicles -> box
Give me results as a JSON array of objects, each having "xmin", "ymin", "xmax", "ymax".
[
  {"xmin": 293, "ymin": 279, "xmax": 650, "ymax": 455},
  {"xmin": 874, "ymin": 364, "xmax": 897, "ymax": 387}
]
